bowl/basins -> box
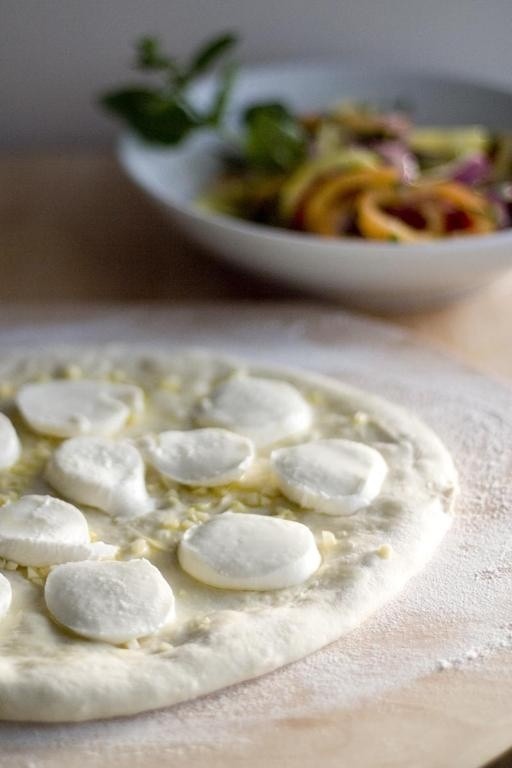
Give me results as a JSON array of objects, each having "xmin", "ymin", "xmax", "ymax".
[{"xmin": 116, "ymin": 52, "xmax": 512, "ymax": 323}]
